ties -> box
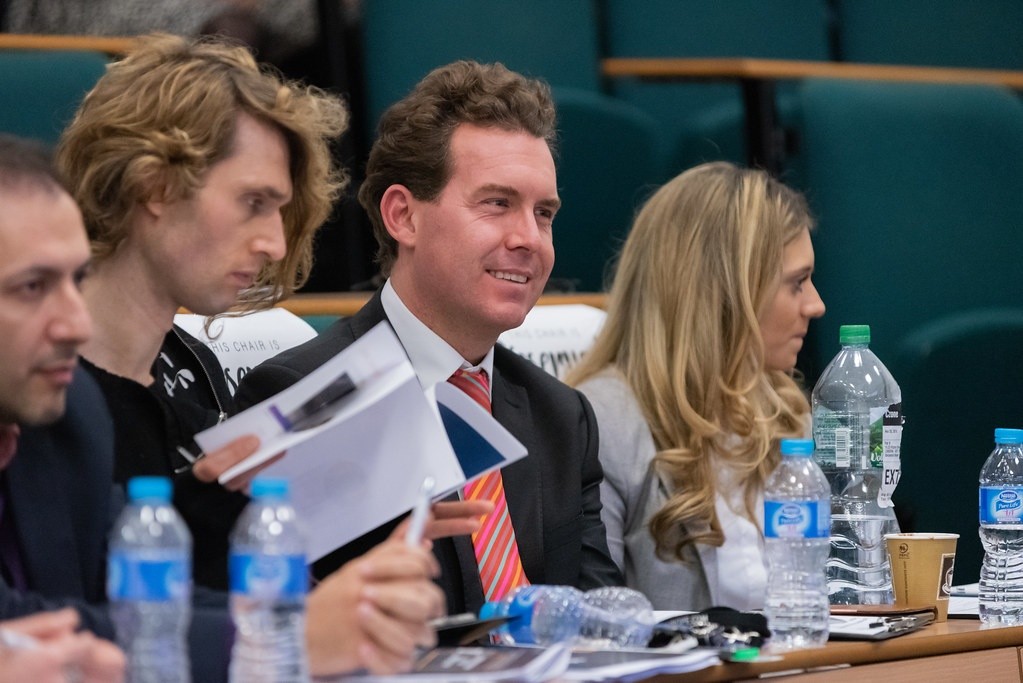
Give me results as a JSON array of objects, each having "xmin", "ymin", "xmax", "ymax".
[{"xmin": 444, "ymin": 370, "xmax": 530, "ymax": 608}]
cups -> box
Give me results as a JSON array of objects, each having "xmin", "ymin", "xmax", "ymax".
[{"xmin": 883, "ymin": 532, "xmax": 959, "ymax": 623}]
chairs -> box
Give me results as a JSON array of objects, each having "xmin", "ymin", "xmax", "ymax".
[{"xmin": 2, "ymin": 4, "xmax": 1023, "ymax": 567}]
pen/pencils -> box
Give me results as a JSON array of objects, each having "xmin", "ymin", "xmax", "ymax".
[
  {"xmin": 403, "ymin": 480, "xmax": 434, "ymax": 548},
  {"xmin": 425, "ymin": 610, "xmax": 478, "ymax": 634}
]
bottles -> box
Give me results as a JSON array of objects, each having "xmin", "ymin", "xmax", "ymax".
[
  {"xmin": 228, "ymin": 482, "xmax": 311, "ymax": 683},
  {"xmin": 812, "ymin": 324, "xmax": 903, "ymax": 610},
  {"xmin": 105, "ymin": 477, "xmax": 194, "ymax": 682},
  {"xmin": 980, "ymin": 429, "xmax": 1023, "ymax": 627},
  {"xmin": 762, "ymin": 437, "xmax": 832, "ymax": 648},
  {"xmin": 478, "ymin": 582, "xmax": 654, "ymax": 648}
]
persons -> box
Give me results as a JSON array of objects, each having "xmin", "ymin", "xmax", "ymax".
[
  {"xmin": 228, "ymin": 57, "xmax": 629, "ymax": 652},
  {"xmin": 556, "ymin": 157, "xmax": 907, "ymax": 618},
  {"xmin": 1, "ymin": 126, "xmax": 132, "ymax": 683},
  {"xmin": 50, "ymin": 31, "xmax": 496, "ymax": 683}
]
